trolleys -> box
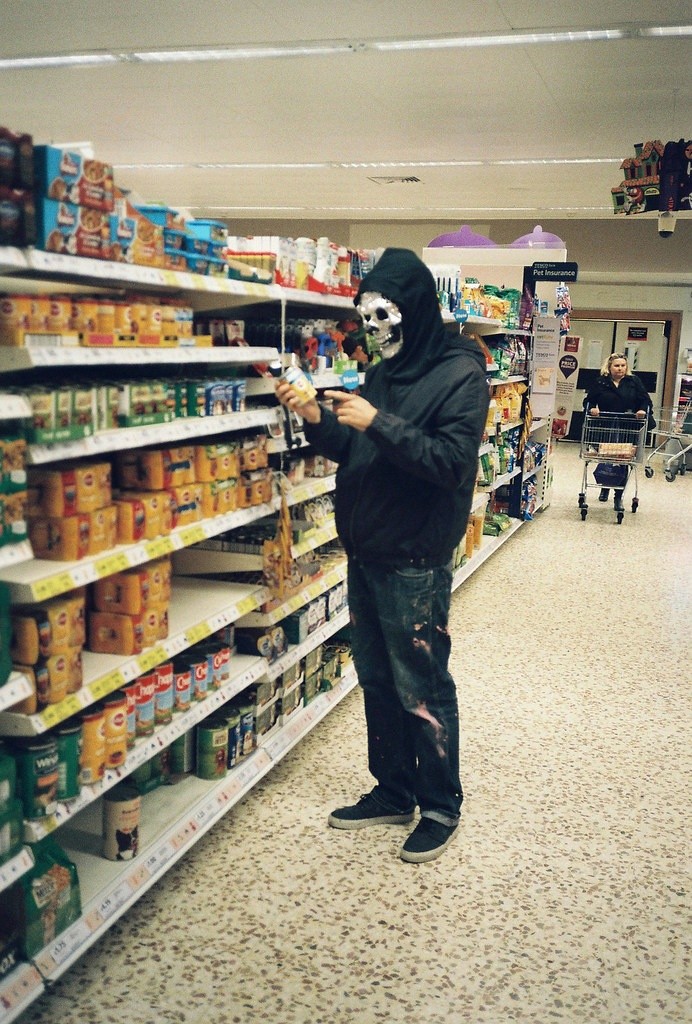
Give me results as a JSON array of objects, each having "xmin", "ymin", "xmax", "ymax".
[
  {"xmin": 646, "ymin": 400, "xmax": 692, "ymax": 482},
  {"xmin": 574, "ymin": 402, "xmax": 652, "ymax": 524}
]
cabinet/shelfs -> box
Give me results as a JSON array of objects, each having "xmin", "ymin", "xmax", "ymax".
[{"xmin": 0, "ymin": 222, "xmax": 578, "ymax": 1023}]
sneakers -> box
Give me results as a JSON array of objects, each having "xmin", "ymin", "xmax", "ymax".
[
  {"xmin": 328, "ymin": 795, "xmax": 413, "ymax": 828},
  {"xmin": 401, "ymin": 816, "xmax": 460, "ymax": 862}
]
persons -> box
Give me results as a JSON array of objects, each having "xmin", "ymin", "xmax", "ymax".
[
  {"xmin": 274, "ymin": 240, "xmax": 493, "ymax": 866},
  {"xmin": 582, "ymin": 350, "xmax": 654, "ymax": 512}
]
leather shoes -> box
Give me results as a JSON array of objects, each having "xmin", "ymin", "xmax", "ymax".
[
  {"xmin": 614, "ymin": 498, "xmax": 627, "ymax": 510},
  {"xmin": 599, "ymin": 490, "xmax": 609, "ymax": 501}
]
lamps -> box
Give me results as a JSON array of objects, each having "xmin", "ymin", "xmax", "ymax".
[
  {"xmin": 112, "ymin": 158, "xmax": 628, "ymax": 171},
  {"xmin": 0, "ymin": 22, "xmax": 692, "ymax": 72}
]
